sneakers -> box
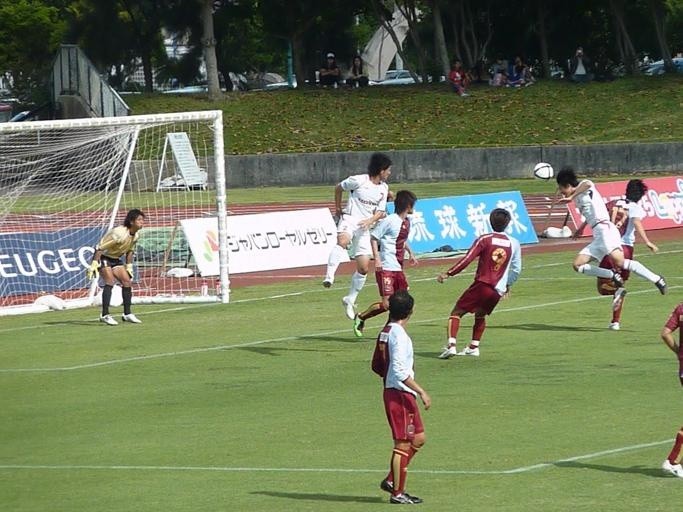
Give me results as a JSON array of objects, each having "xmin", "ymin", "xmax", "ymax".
[
  {"xmin": 612, "ymin": 288, "xmax": 626, "ymax": 311},
  {"xmin": 655, "ymin": 274, "xmax": 667, "ymax": 295},
  {"xmin": 456, "ymin": 347, "xmax": 480, "ymax": 356},
  {"xmin": 609, "ymin": 323, "xmax": 620, "ymax": 331},
  {"xmin": 100, "ymin": 314, "xmax": 119, "ymax": 325},
  {"xmin": 662, "ymin": 459, "xmax": 683, "ymax": 479},
  {"xmin": 343, "ymin": 296, "xmax": 355, "ymax": 320},
  {"xmin": 122, "ymin": 313, "xmax": 141, "ymax": 323},
  {"xmin": 438, "ymin": 346, "xmax": 456, "ymax": 358},
  {"xmin": 322, "ymin": 280, "xmax": 331, "ymax": 288},
  {"xmin": 390, "ymin": 494, "xmax": 422, "ymax": 504},
  {"xmin": 381, "ymin": 480, "xmax": 394, "ymax": 493},
  {"xmin": 612, "ymin": 268, "xmax": 623, "ymax": 287},
  {"xmin": 353, "ymin": 313, "xmax": 364, "ymax": 337}
]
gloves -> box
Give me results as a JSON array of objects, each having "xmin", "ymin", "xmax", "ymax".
[
  {"xmin": 88, "ymin": 261, "xmax": 98, "ymax": 280},
  {"xmin": 125, "ymin": 264, "xmax": 133, "ymax": 278}
]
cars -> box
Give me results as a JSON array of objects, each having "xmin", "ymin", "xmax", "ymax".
[
  {"xmin": 2, "ymin": 98, "xmax": 43, "ymax": 125},
  {"xmin": 111, "ymin": 68, "xmax": 424, "ymax": 96},
  {"xmin": 640, "ymin": 57, "xmax": 682, "ymax": 75}
]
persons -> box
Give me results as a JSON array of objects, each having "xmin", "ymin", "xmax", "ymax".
[
  {"xmin": 437, "ymin": 209, "xmax": 522, "ymax": 358},
  {"xmin": 555, "ymin": 169, "xmax": 668, "ymax": 296},
  {"xmin": 318, "ymin": 52, "xmax": 340, "ymax": 89},
  {"xmin": 348, "ymin": 57, "xmax": 368, "ymax": 90},
  {"xmin": 88, "ymin": 209, "xmax": 145, "ymax": 325},
  {"xmin": 371, "ymin": 289, "xmax": 431, "ymax": 505},
  {"xmin": 596, "ymin": 179, "xmax": 659, "ymax": 330},
  {"xmin": 569, "ymin": 47, "xmax": 595, "ymax": 82},
  {"xmin": 488, "ymin": 54, "xmax": 510, "ymax": 88},
  {"xmin": 322, "ymin": 153, "xmax": 393, "ymax": 320},
  {"xmin": 510, "ymin": 57, "xmax": 536, "ymax": 89},
  {"xmin": 354, "ymin": 190, "xmax": 419, "ymax": 338},
  {"xmin": 449, "ymin": 60, "xmax": 470, "ymax": 97},
  {"xmin": 660, "ymin": 303, "xmax": 683, "ymax": 478}
]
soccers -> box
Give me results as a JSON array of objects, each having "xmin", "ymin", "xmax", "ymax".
[{"xmin": 534, "ymin": 162, "xmax": 553, "ymax": 180}]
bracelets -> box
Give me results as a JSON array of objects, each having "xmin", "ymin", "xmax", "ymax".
[{"xmin": 335, "ymin": 208, "xmax": 341, "ymax": 210}]
